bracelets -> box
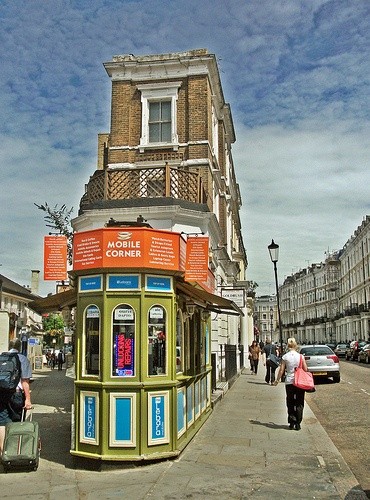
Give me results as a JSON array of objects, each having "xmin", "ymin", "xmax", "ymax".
[{"xmin": 276, "ymin": 379, "xmax": 279, "ymax": 382}]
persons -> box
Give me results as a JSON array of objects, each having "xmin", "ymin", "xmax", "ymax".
[
  {"xmin": 58, "ymin": 350, "xmax": 65, "ymax": 371},
  {"xmin": 300, "ymin": 340, "xmax": 325, "ymax": 345},
  {"xmin": 350, "ymin": 339, "xmax": 356, "ymax": 346},
  {"xmin": 355, "ymin": 338, "xmax": 370, "ymax": 350},
  {"xmin": 46, "ymin": 350, "xmax": 55, "ymax": 370},
  {"xmin": 338, "ymin": 339, "xmax": 354, "ymax": 344},
  {"xmin": 259, "ymin": 340, "xmax": 264, "ymax": 354},
  {"xmin": 248, "ymin": 340, "xmax": 259, "ymax": 374},
  {"xmin": 272, "ymin": 337, "xmax": 308, "ymax": 430},
  {"xmin": 0, "ymin": 338, "xmax": 33, "ymax": 462},
  {"xmin": 264, "ymin": 339, "xmax": 279, "ymax": 385}
]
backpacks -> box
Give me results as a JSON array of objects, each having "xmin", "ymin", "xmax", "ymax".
[{"xmin": 0, "ymin": 351, "xmax": 22, "ymax": 393}]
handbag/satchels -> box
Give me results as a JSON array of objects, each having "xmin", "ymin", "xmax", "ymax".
[
  {"xmin": 292, "ymin": 367, "xmax": 314, "ymax": 391},
  {"xmin": 266, "ymin": 354, "xmax": 280, "ymax": 369}
]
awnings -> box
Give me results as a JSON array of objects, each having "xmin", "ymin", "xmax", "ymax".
[
  {"xmin": 176, "ymin": 281, "xmax": 245, "ymax": 316},
  {"xmin": 25, "ymin": 289, "xmax": 77, "ymax": 316}
]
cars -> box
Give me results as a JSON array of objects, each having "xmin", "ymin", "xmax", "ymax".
[
  {"xmin": 357, "ymin": 343, "xmax": 370, "ymax": 364},
  {"xmin": 296, "ymin": 345, "xmax": 340, "ymax": 383},
  {"xmin": 323, "ymin": 343, "xmax": 336, "ymax": 351},
  {"xmin": 333, "ymin": 343, "xmax": 350, "ymax": 358},
  {"xmin": 344, "ymin": 340, "xmax": 367, "ymax": 360}
]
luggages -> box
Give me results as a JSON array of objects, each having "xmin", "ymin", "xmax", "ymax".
[{"xmin": 1, "ymin": 407, "xmax": 43, "ymax": 473}]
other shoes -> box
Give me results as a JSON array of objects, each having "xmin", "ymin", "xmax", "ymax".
[
  {"xmin": 288, "ymin": 422, "xmax": 294, "ymax": 430},
  {"xmin": 295, "ymin": 422, "xmax": 302, "ymax": 431}
]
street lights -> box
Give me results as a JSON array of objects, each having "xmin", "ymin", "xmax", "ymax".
[
  {"xmin": 267, "ymin": 238, "xmax": 286, "ymax": 382},
  {"xmin": 269, "ymin": 308, "xmax": 274, "ymax": 344}
]
